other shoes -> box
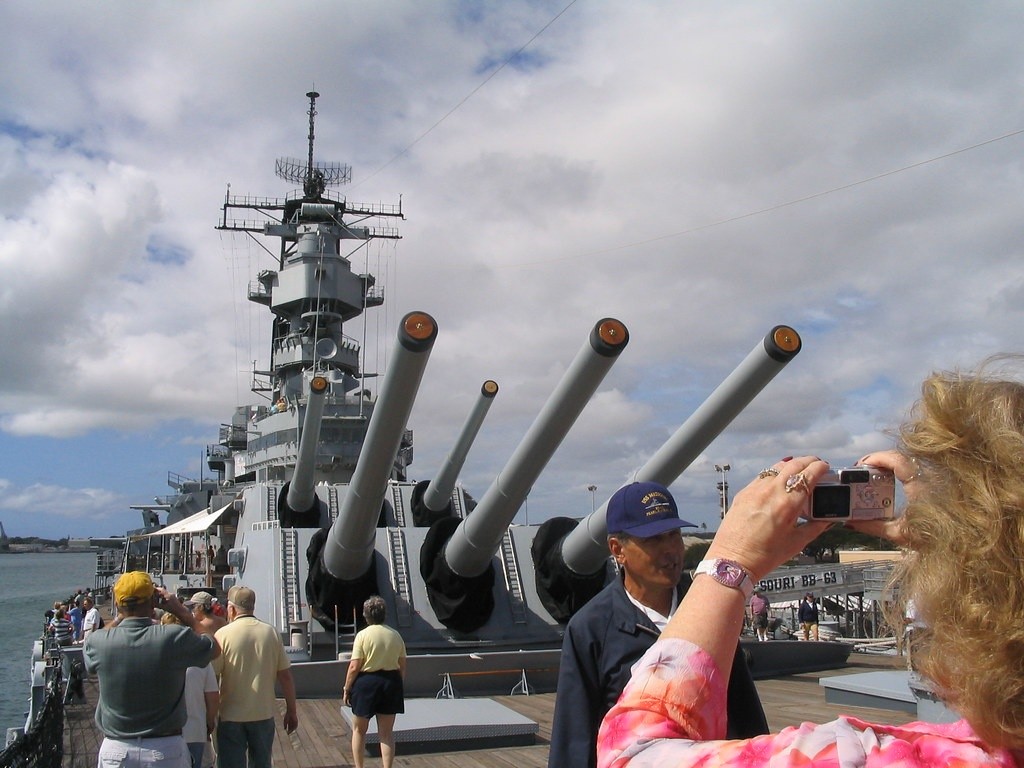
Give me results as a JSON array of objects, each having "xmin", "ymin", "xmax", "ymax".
[
  {"xmin": 759, "ymin": 638, "xmax": 763, "ymax": 642},
  {"xmin": 764, "ymin": 637, "xmax": 769, "ymax": 641}
]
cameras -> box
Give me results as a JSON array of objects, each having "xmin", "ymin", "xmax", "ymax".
[
  {"xmin": 152, "ymin": 594, "xmax": 159, "ymax": 607},
  {"xmin": 800, "ymin": 466, "xmax": 896, "ymax": 521}
]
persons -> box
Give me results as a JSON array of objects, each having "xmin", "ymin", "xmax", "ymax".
[
  {"xmin": 596, "ymin": 366, "xmax": 1023, "ymax": 765},
  {"xmin": 343, "ymin": 597, "xmax": 407, "ymax": 768},
  {"xmin": 270, "ymin": 397, "xmax": 288, "ymax": 414},
  {"xmin": 149, "ymin": 551, "xmax": 168, "ymax": 569},
  {"xmin": 195, "ymin": 550, "xmax": 202, "ymax": 568},
  {"xmin": 151, "ymin": 580, "xmax": 300, "ymax": 767},
  {"xmin": 207, "ymin": 545, "xmax": 215, "ymax": 563},
  {"xmin": 82, "ymin": 571, "xmax": 221, "ymax": 768},
  {"xmin": 549, "ymin": 482, "xmax": 771, "ymax": 768},
  {"xmin": 749, "ymin": 587, "xmax": 771, "ymax": 642},
  {"xmin": 46, "ymin": 584, "xmax": 104, "ymax": 655},
  {"xmin": 798, "ymin": 591, "xmax": 819, "ymax": 642}
]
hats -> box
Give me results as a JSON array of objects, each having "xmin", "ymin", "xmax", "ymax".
[
  {"xmin": 806, "ymin": 591, "xmax": 814, "ymax": 598},
  {"xmin": 114, "ymin": 571, "xmax": 154, "ymax": 606},
  {"xmin": 227, "ymin": 585, "xmax": 256, "ymax": 610},
  {"xmin": 753, "ymin": 586, "xmax": 762, "ymax": 592},
  {"xmin": 183, "ymin": 591, "xmax": 213, "ymax": 606},
  {"xmin": 605, "ymin": 481, "xmax": 699, "ymax": 538}
]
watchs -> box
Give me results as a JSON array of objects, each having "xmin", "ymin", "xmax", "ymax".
[{"xmin": 689, "ymin": 557, "xmax": 754, "ymax": 600}]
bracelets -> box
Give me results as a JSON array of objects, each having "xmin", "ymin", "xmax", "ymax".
[{"xmin": 341, "ymin": 685, "xmax": 348, "ymax": 694}]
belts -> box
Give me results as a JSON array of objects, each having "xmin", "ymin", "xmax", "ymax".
[{"xmin": 104, "ymin": 729, "xmax": 183, "ymax": 740}]
